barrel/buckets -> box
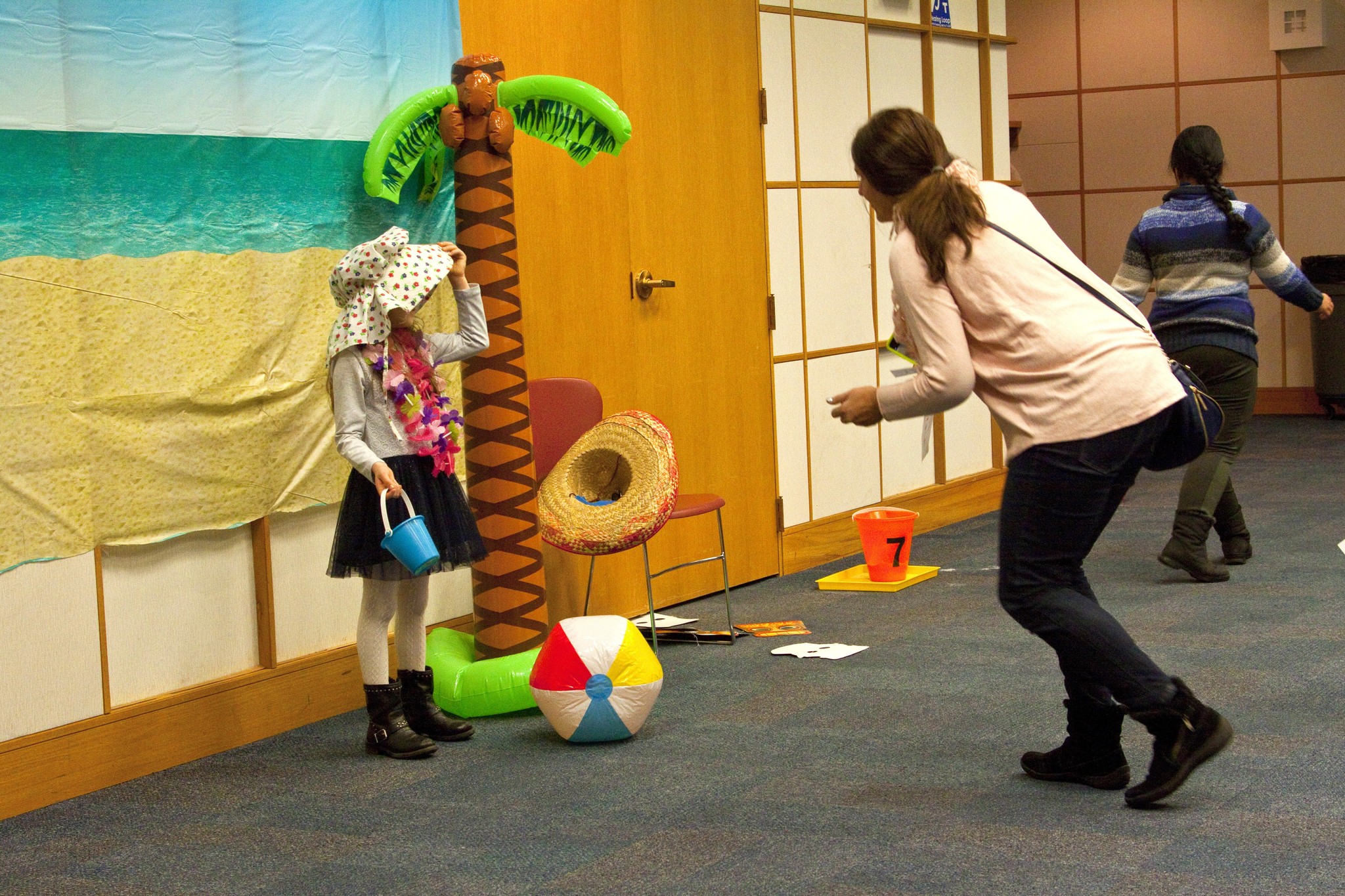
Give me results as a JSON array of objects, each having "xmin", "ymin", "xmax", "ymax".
[
  {"xmin": 852, "ymin": 507, "xmax": 920, "ymax": 582},
  {"xmin": 379, "ymin": 485, "xmax": 441, "ymax": 573}
]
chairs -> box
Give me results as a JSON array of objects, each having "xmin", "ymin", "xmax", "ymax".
[{"xmin": 526, "ymin": 377, "xmax": 735, "ymax": 659}]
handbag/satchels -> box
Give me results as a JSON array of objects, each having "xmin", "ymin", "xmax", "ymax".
[{"xmin": 1142, "ymin": 359, "xmax": 1227, "ymax": 471}]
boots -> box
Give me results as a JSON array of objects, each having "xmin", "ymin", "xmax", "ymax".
[
  {"xmin": 1021, "ymin": 697, "xmax": 1132, "ymax": 790},
  {"xmin": 363, "ymin": 676, "xmax": 440, "ymax": 759},
  {"xmin": 1124, "ymin": 677, "xmax": 1233, "ymax": 806},
  {"xmin": 1213, "ymin": 504, "xmax": 1252, "ymax": 563},
  {"xmin": 1158, "ymin": 509, "xmax": 1231, "ymax": 583},
  {"xmin": 397, "ymin": 665, "xmax": 475, "ymax": 740}
]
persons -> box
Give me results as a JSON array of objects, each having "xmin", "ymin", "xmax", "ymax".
[
  {"xmin": 325, "ymin": 226, "xmax": 491, "ymax": 759},
  {"xmin": 1110, "ymin": 125, "xmax": 1336, "ymax": 584},
  {"xmin": 826, "ymin": 106, "xmax": 1233, "ymax": 806}
]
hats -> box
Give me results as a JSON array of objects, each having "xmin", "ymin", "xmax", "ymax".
[
  {"xmin": 324, "ymin": 225, "xmax": 455, "ymax": 360},
  {"xmin": 537, "ymin": 409, "xmax": 680, "ymax": 555}
]
